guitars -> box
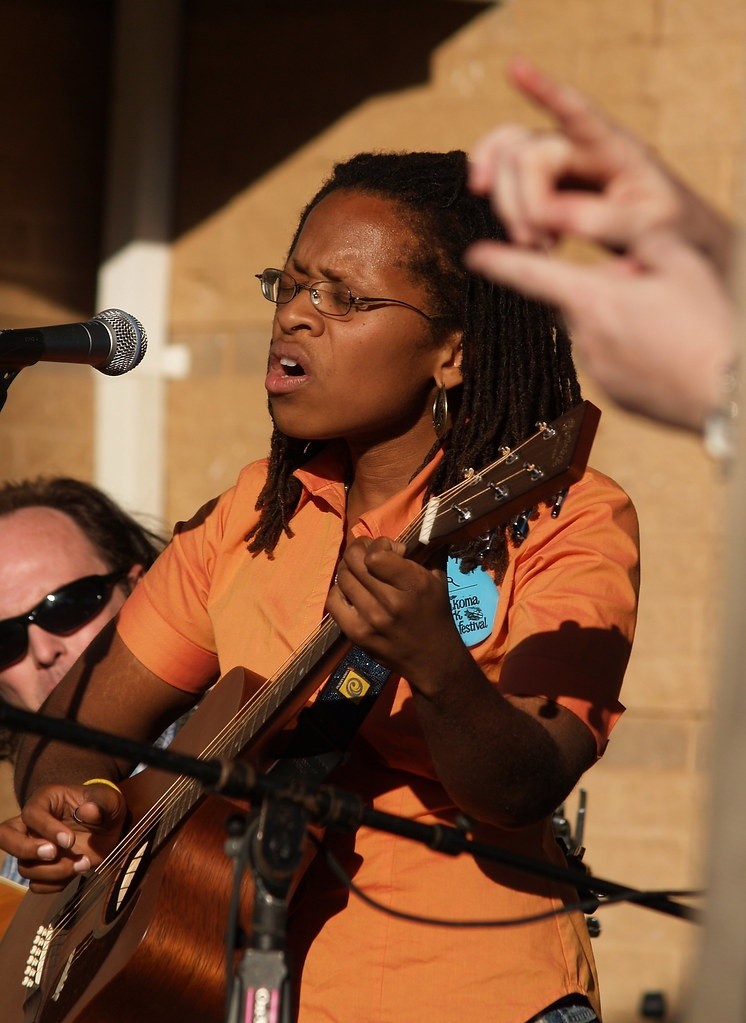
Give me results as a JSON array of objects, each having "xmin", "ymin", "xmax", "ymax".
[{"xmin": 0, "ymin": 395, "xmax": 606, "ymax": 1023}]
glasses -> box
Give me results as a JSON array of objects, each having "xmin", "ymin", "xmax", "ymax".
[
  {"xmin": 0, "ymin": 568, "xmax": 125, "ymax": 667},
  {"xmin": 255, "ymin": 268, "xmax": 451, "ymax": 322}
]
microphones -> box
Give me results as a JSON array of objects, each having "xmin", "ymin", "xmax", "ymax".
[{"xmin": 0, "ymin": 309, "xmax": 148, "ymax": 376}]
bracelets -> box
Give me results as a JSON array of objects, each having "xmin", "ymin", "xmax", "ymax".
[
  {"xmin": 82, "ymin": 778, "xmax": 122, "ymax": 794},
  {"xmin": 702, "ymin": 353, "xmax": 746, "ymax": 481}
]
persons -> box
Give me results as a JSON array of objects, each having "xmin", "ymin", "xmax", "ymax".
[
  {"xmin": 466, "ymin": 56, "xmax": 745, "ymax": 461},
  {"xmin": 1, "ymin": 150, "xmax": 641, "ymax": 1023},
  {"xmin": 0, "ymin": 473, "xmax": 205, "ymax": 890}
]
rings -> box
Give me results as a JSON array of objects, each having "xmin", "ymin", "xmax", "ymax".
[{"xmin": 73, "ymin": 807, "xmax": 84, "ymax": 824}]
